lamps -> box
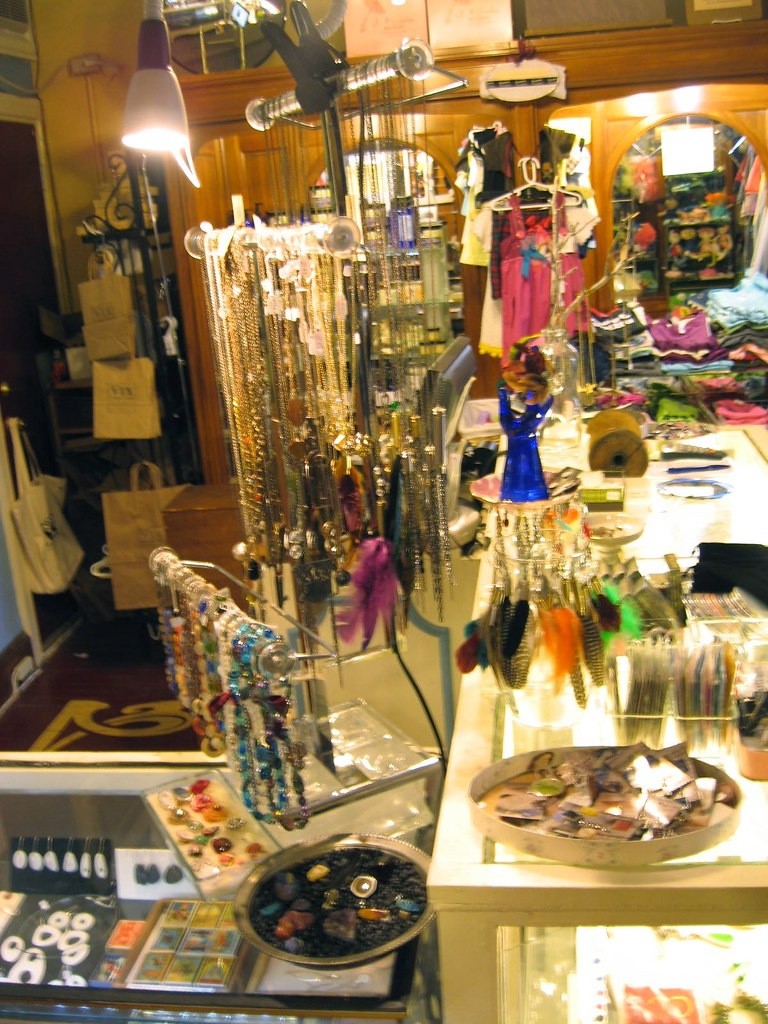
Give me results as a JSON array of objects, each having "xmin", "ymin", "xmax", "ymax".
[{"xmin": 121, "ymin": 0, "xmax": 191, "ymax": 153}]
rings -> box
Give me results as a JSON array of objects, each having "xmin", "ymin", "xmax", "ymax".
[{"xmin": 157, "ymin": 779, "xmax": 262, "ymax": 882}]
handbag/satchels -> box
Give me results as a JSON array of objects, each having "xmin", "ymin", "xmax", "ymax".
[
  {"xmin": 91, "ymin": 357, "xmax": 163, "ymax": 441},
  {"xmin": 100, "ymin": 460, "xmax": 196, "ymax": 610},
  {"xmin": 74, "ymin": 250, "xmax": 137, "ymax": 364},
  {"xmin": 7, "ymin": 418, "xmax": 84, "ymax": 595}
]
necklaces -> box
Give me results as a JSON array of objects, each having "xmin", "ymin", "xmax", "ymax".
[
  {"xmin": 258, "ymin": 55, "xmax": 456, "ymax": 630},
  {"xmin": 152, "ymin": 554, "xmax": 310, "ymax": 831},
  {"xmin": 576, "ymin": 256, "xmax": 638, "ymax": 407},
  {"xmin": 13, "ymin": 836, "xmax": 108, "ymax": 879},
  {"xmin": 198, "ymin": 224, "xmax": 353, "ymax": 552}
]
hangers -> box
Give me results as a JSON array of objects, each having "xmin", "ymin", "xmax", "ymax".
[{"xmin": 489, "ymin": 155, "xmax": 582, "ymax": 211}]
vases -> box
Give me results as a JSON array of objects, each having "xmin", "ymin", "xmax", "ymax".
[{"xmin": 529, "ymin": 330, "xmax": 582, "ymax": 449}]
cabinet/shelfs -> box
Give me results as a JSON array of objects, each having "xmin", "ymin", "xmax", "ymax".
[{"xmin": 37, "ymin": 155, "xmax": 204, "ymax": 658}]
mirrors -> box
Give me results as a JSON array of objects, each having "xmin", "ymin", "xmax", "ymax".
[
  {"xmin": 164, "ymin": 0, "xmax": 286, "ymax": 75},
  {"xmin": 609, "ymin": 111, "xmax": 764, "ymax": 295},
  {"xmin": 303, "ymin": 139, "xmax": 467, "ymax": 401}
]
